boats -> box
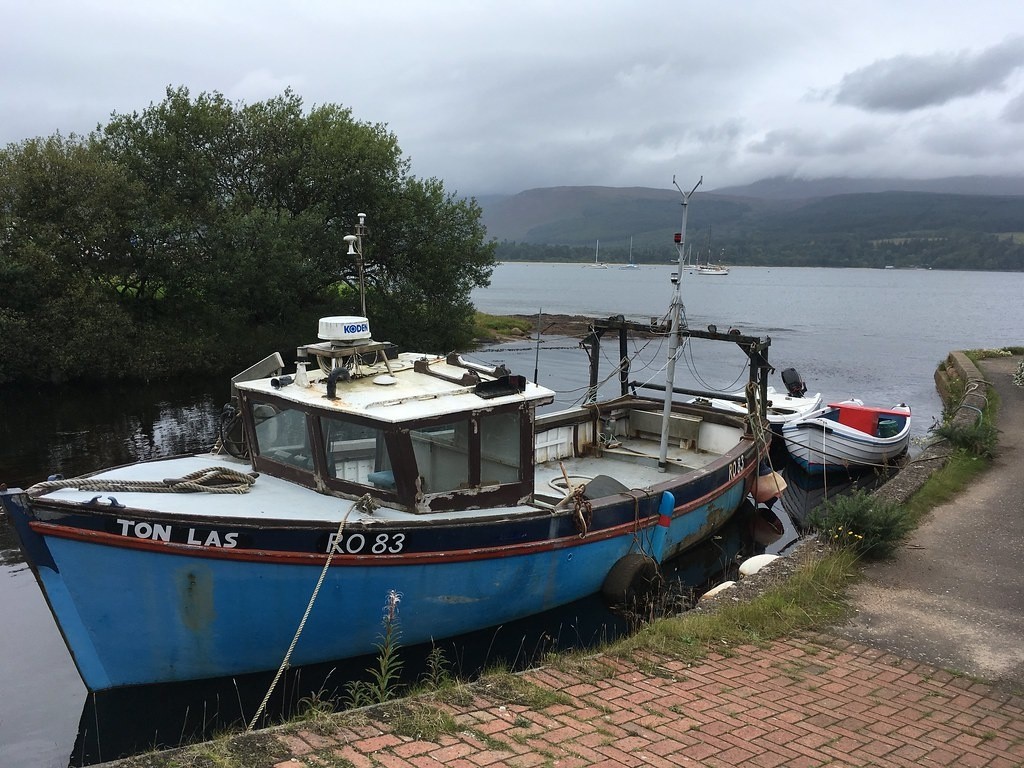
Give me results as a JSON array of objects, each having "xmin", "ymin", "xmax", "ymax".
[
  {"xmin": 1, "ymin": 209, "xmax": 773, "ymax": 697},
  {"xmin": 780, "ymin": 397, "xmax": 913, "ymax": 474},
  {"xmin": 688, "ymin": 385, "xmax": 823, "ymax": 456}
]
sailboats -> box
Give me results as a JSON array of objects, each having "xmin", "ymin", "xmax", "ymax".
[
  {"xmin": 590, "ymin": 239, "xmax": 610, "ymax": 269},
  {"xmin": 617, "ymin": 234, "xmax": 641, "ymax": 271},
  {"xmin": 684, "ymin": 222, "xmax": 730, "ymax": 275}
]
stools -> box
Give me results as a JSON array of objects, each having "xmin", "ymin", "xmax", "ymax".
[{"xmin": 367, "ymin": 471, "xmax": 397, "ymax": 492}]
